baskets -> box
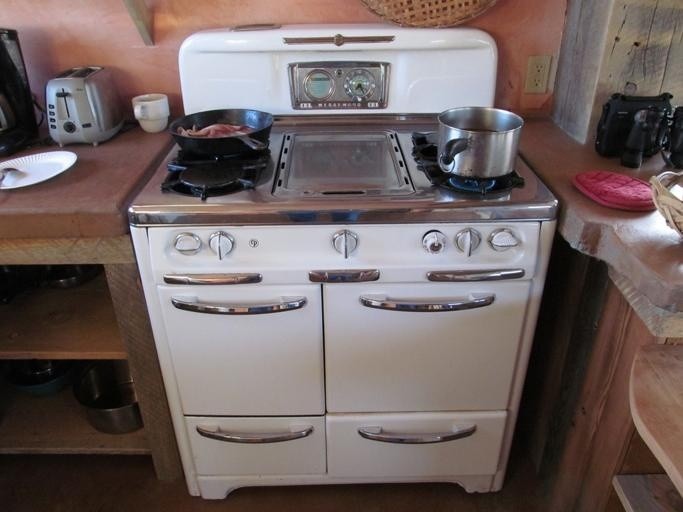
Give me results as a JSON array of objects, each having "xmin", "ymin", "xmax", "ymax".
[
  {"xmin": 649, "ymin": 170, "xmax": 682, "ymax": 235},
  {"xmin": 359, "ymin": 0, "xmax": 496, "ymax": 29}
]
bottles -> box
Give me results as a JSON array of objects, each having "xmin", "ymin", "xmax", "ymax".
[{"xmin": 622, "ymin": 122, "xmax": 647, "ymax": 168}]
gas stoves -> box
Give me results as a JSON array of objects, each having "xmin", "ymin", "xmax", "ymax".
[{"xmin": 131, "ymin": 114, "xmax": 555, "ymax": 208}]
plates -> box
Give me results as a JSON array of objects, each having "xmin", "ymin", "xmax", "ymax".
[{"xmin": 0, "ymin": 151, "xmax": 78, "ymax": 190}]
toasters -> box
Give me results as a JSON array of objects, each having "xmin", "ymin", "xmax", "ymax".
[{"xmin": 44, "ymin": 66, "xmax": 125, "ymax": 148}]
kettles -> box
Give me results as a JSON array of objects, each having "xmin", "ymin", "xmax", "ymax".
[{"xmin": 0, "ymin": 27, "xmax": 39, "ymax": 156}]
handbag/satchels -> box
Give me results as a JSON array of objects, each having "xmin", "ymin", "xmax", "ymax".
[{"xmin": 595, "ymin": 92, "xmax": 673, "ymax": 159}]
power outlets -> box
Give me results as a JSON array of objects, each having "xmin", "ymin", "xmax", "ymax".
[{"xmin": 524, "ymin": 55, "xmax": 552, "ymax": 93}]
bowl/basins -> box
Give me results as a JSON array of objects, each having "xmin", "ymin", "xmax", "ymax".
[
  {"xmin": 48, "ymin": 276, "xmax": 79, "ymax": 288},
  {"xmin": 71, "ymin": 363, "xmax": 143, "ymax": 435}
]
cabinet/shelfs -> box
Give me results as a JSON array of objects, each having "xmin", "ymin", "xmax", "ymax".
[
  {"xmin": 612, "ymin": 344, "xmax": 682, "ymax": 512},
  {"xmin": 1, "ymin": 122, "xmax": 183, "ymax": 481},
  {"xmin": 142, "ymin": 283, "xmax": 326, "ymax": 499},
  {"xmin": 322, "ymin": 284, "xmax": 544, "ymax": 493}
]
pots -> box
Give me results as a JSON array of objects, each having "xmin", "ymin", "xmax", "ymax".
[
  {"xmin": 170, "ymin": 107, "xmax": 273, "ymax": 158},
  {"xmin": 7, "ymin": 360, "xmax": 70, "ymax": 393},
  {"xmin": 436, "ymin": 107, "xmax": 524, "ymax": 177}
]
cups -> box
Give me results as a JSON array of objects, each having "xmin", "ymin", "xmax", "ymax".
[
  {"xmin": 132, "ymin": 93, "xmax": 171, "ymax": 133},
  {"xmin": 656, "ymin": 106, "xmax": 683, "ymax": 170}
]
